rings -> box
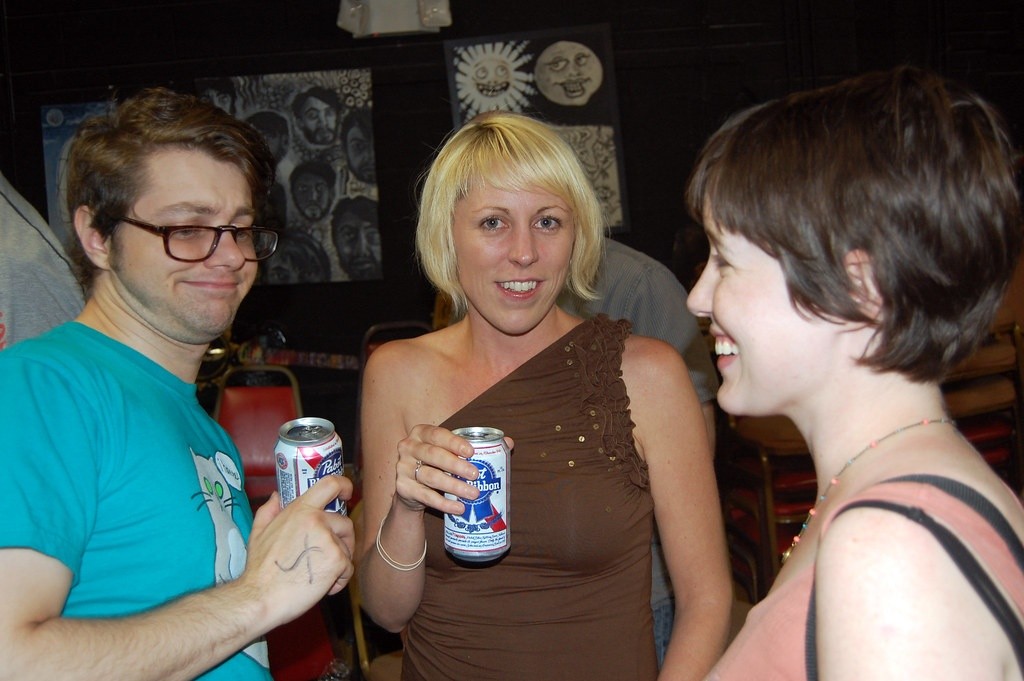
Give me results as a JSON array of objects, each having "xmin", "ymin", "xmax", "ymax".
[{"xmin": 415, "ymin": 461, "xmax": 422, "ymax": 481}]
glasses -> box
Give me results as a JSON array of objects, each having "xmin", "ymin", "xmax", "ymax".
[{"xmin": 102, "ymin": 209, "xmax": 280, "ymax": 262}]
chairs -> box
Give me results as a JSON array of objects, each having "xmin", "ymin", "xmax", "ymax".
[
  {"xmin": 714, "ymin": 422, "xmax": 811, "ymax": 613},
  {"xmin": 214, "ymin": 363, "xmax": 304, "ymax": 503},
  {"xmin": 350, "ymin": 498, "xmax": 404, "ymax": 681}
]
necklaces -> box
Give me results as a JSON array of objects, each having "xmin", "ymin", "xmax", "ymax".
[{"xmin": 780, "ymin": 417, "xmax": 956, "ymax": 562}]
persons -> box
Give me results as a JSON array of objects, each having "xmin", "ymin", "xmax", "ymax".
[
  {"xmin": 195, "ymin": 80, "xmax": 342, "ymax": 283},
  {"xmin": 341, "ymin": 107, "xmax": 375, "ymax": 183},
  {"xmin": 332, "ymin": 196, "xmax": 384, "ymax": 280},
  {"xmin": 0, "ymin": 86, "xmax": 356, "ymax": 681},
  {"xmin": 687, "ymin": 64, "xmax": 1024, "ymax": 680},
  {"xmin": 0, "ymin": 170, "xmax": 88, "ymax": 353},
  {"xmin": 357, "ymin": 110, "xmax": 735, "ymax": 681}
]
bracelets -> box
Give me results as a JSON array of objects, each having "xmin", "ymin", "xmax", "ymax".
[{"xmin": 376, "ymin": 515, "xmax": 428, "ymax": 571}]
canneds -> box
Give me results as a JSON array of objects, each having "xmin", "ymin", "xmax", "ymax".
[
  {"xmin": 273, "ymin": 416, "xmax": 348, "ymax": 517},
  {"xmin": 442, "ymin": 426, "xmax": 510, "ymax": 562}
]
